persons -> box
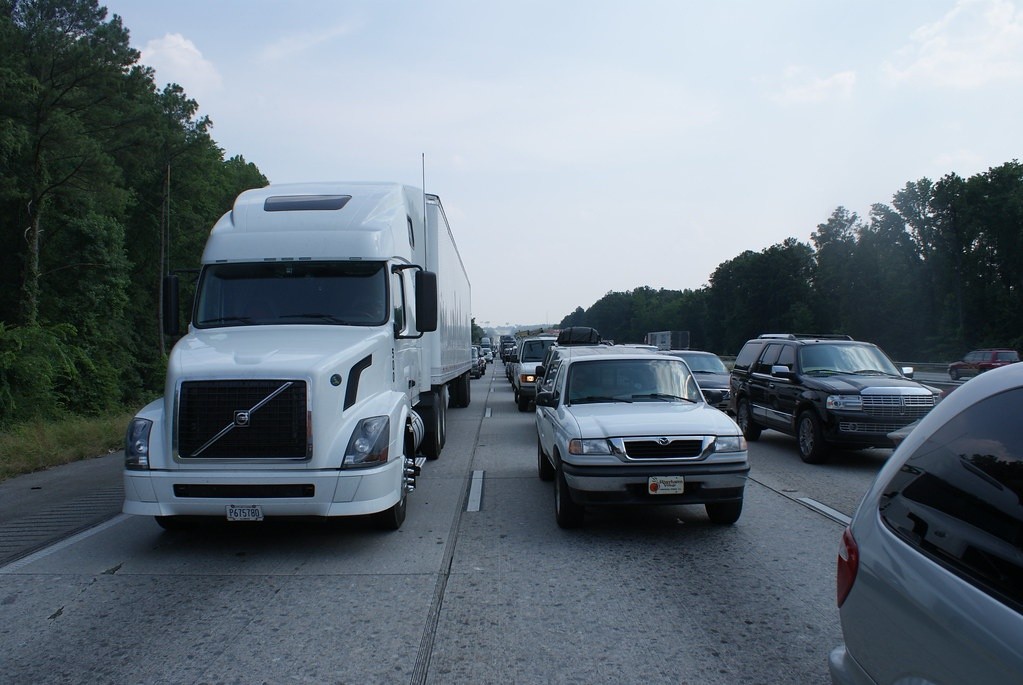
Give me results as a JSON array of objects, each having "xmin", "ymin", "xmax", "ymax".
[{"xmin": 571, "ymin": 369, "xmax": 590, "ymax": 399}]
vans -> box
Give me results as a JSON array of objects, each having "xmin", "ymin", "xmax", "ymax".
[{"xmin": 510, "ymin": 336, "xmax": 558, "ymax": 411}]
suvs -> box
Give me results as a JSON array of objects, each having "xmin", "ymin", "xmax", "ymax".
[
  {"xmin": 947, "ymin": 349, "xmax": 1020, "ymax": 380},
  {"xmin": 729, "ymin": 333, "xmax": 945, "ymax": 464},
  {"xmin": 536, "ymin": 324, "xmax": 752, "ymax": 526}
]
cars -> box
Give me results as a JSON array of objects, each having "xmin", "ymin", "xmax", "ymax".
[
  {"xmin": 479, "ymin": 334, "xmax": 499, "ymax": 357},
  {"xmin": 483, "ymin": 348, "xmax": 493, "ymax": 364},
  {"xmin": 499, "ymin": 327, "xmax": 614, "ymax": 382},
  {"xmin": 828, "ymin": 360, "xmax": 1023, "ymax": 684},
  {"xmin": 655, "ymin": 350, "xmax": 731, "ymax": 417},
  {"xmin": 614, "ymin": 342, "xmax": 659, "ymax": 350},
  {"xmin": 471, "ymin": 345, "xmax": 486, "ymax": 375},
  {"xmin": 471, "ymin": 347, "xmax": 481, "ymax": 380}
]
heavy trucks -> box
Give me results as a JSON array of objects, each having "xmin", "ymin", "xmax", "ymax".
[{"xmin": 121, "ymin": 150, "xmax": 472, "ymax": 531}]
